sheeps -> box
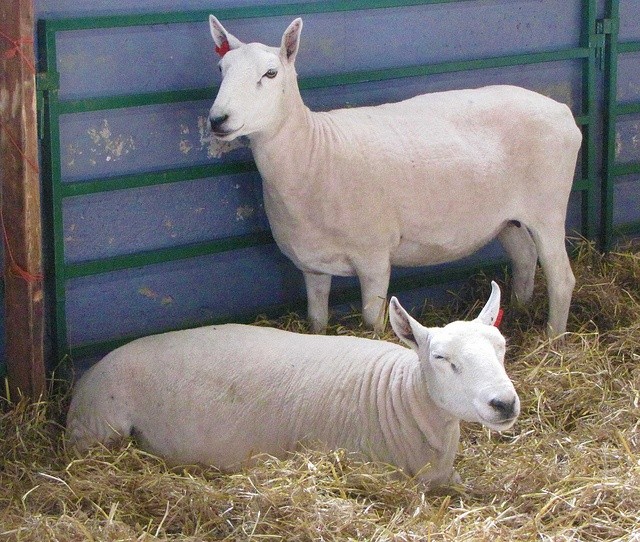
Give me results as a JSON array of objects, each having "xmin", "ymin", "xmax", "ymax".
[
  {"xmin": 204, "ymin": 13, "xmax": 585, "ymax": 341},
  {"xmin": 68, "ymin": 281, "xmax": 521, "ymax": 493}
]
